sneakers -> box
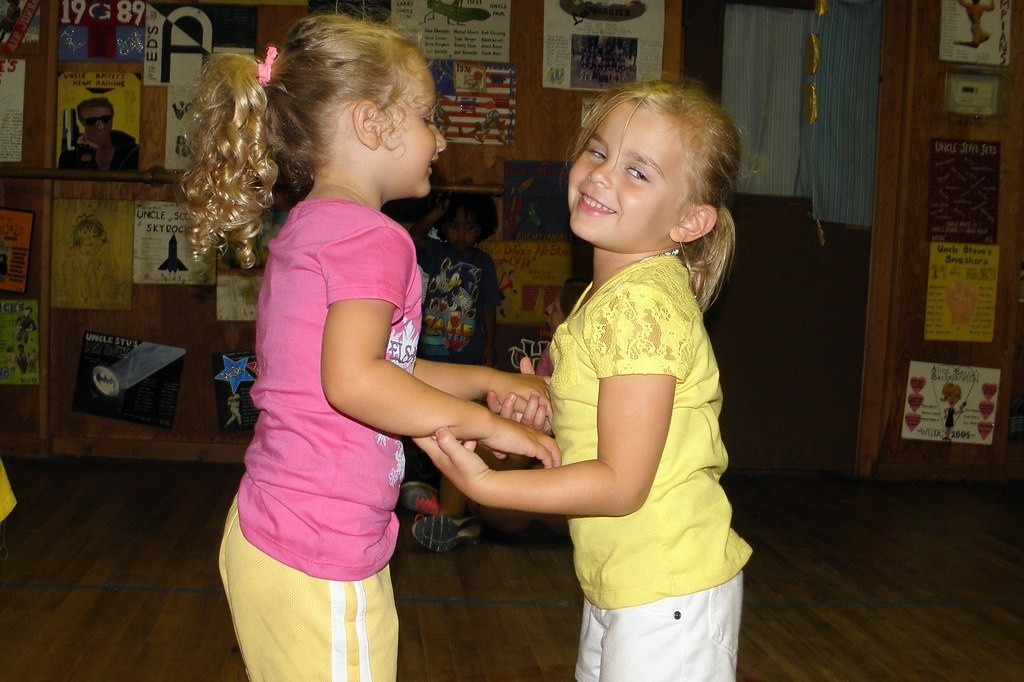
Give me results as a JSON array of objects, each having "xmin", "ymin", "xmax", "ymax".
[
  {"xmin": 411, "ymin": 513, "xmax": 488, "ymax": 552},
  {"xmin": 399, "ymin": 481, "xmax": 442, "ymax": 516}
]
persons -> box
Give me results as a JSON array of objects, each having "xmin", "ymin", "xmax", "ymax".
[
  {"xmin": 403, "ymin": 192, "xmax": 501, "ymax": 512},
  {"xmin": 182, "ymin": 13, "xmax": 559, "ymax": 679},
  {"xmin": 414, "ymin": 75, "xmax": 755, "ymax": 682},
  {"xmin": 411, "ymin": 279, "xmax": 588, "ymax": 550},
  {"xmin": 57, "ymin": 98, "xmax": 138, "ymax": 170}
]
